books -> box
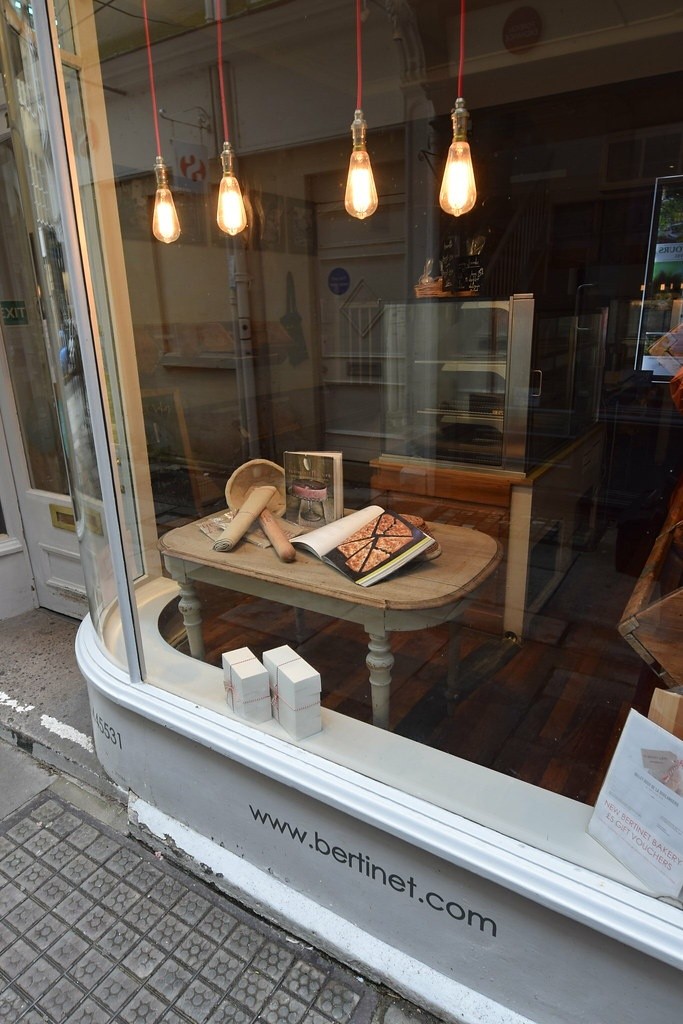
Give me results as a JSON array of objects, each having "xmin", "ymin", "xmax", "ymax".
[
  {"xmin": 283, "ymin": 451, "xmax": 344, "ymax": 530},
  {"xmin": 288, "ymin": 505, "xmax": 435, "ymax": 588}
]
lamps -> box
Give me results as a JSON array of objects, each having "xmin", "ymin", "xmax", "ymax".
[
  {"xmin": 439, "ymin": 0, "xmax": 477, "ymax": 216},
  {"xmin": 344, "ymin": 0, "xmax": 379, "ymax": 220},
  {"xmin": 142, "ymin": 0, "xmax": 181, "ymax": 244},
  {"xmin": 213, "ymin": 0, "xmax": 246, "ymax": 237}
]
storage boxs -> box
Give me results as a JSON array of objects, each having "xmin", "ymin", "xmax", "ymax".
[
  {"xmin": 261, "ymin": 643, "xmax": 323, "ymax": 742},
  {"xmin": 221, "ymin": 647, "xmax": 272, "ymax": 724}
]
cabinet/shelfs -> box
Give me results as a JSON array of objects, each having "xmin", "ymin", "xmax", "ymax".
[
  {"xmin": 377, "ymin": 287, "xmax": 610, "ymax": 475},
  {"xmin": 371, "ymin": 419, "xmax": 606, "ymax": 646}
]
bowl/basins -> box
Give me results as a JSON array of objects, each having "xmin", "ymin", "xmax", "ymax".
[{"xmin": 224, "ymin": 458, "xmax": 285, "ymax": 520}]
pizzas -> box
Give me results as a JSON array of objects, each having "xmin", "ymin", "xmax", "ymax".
[{"xmin": 337, "ymin": 514, "xmax": 414, "ymax": 573}]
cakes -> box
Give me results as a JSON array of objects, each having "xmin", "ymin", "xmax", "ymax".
[{"xmin": 291, "ymin": 479, "xmax": 327, "ymax": 500}]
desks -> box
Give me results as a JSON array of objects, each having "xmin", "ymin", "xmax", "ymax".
[{"xmin": 156, "ymin": 508, "xmax": 502, "ymax": 730}]
